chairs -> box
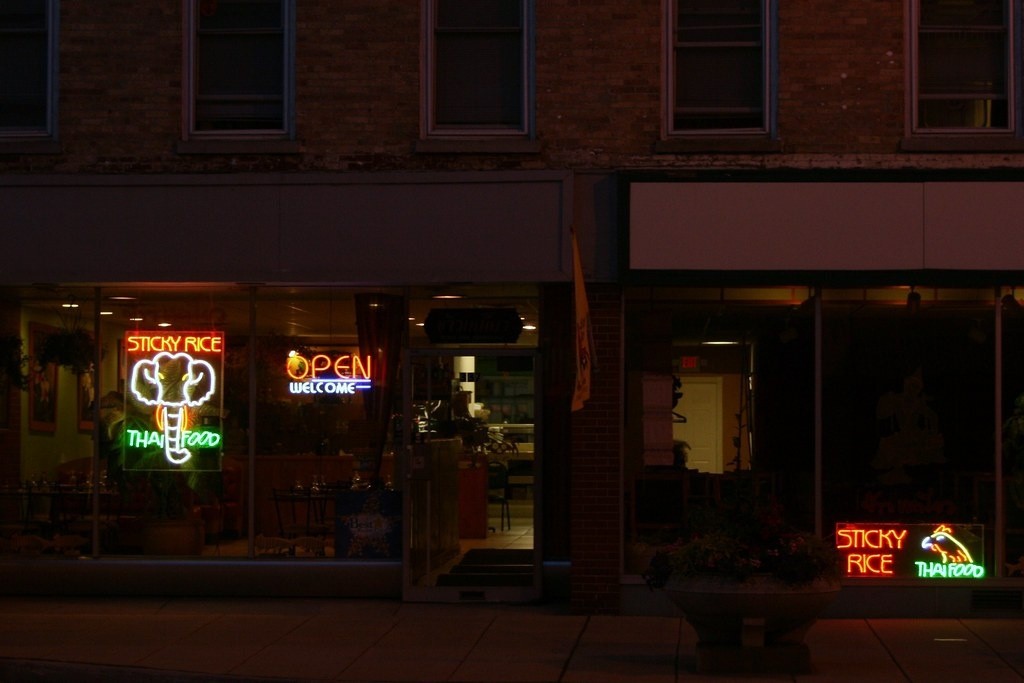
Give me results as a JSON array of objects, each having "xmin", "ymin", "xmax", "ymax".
[
  {"xmin": 18, "ymin": 481, "xmax": 61, "ymax": 560},
  {"xmin": 483, "ymin": 462, "xmax": 513, "ymax": 532},
  {"xmin": 273, "ymin": 482, "xmax": 342, "ymax": 556}
]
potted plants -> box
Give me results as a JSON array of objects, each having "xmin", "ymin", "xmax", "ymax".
[{"xmin": 110, "ymin": 410, "xmax": 224, "ymax": 554}]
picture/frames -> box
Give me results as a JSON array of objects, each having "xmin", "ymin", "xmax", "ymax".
[{"xmin": 21, "ymin": 320, "xmax": 59, "ymax": 434}]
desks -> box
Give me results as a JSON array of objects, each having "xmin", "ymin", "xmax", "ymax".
[
  {"xmin": 15, "ymin": 490, "xmax": 116, "ymax": 553},
  {"xmin": 279, "ymin": 493, "xmax": 341, "ymax": 556}
]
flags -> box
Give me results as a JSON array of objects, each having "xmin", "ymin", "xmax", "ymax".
[{"xmin": 569, "ymin": 232, "xmax": 592, "ymax": 412}]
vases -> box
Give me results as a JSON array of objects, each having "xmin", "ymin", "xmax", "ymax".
[
  {"xmin": 74, "ymin": 329, "xmax": 103, "ymax": 432},
  {"xmin": 649, "ymin": 574, "xmax": 842, "ymax": 674}
]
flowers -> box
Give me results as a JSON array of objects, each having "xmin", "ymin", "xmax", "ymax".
[{"xmin": 627, "ymin": 500, "xmax": 844, "ymax": 584}]
